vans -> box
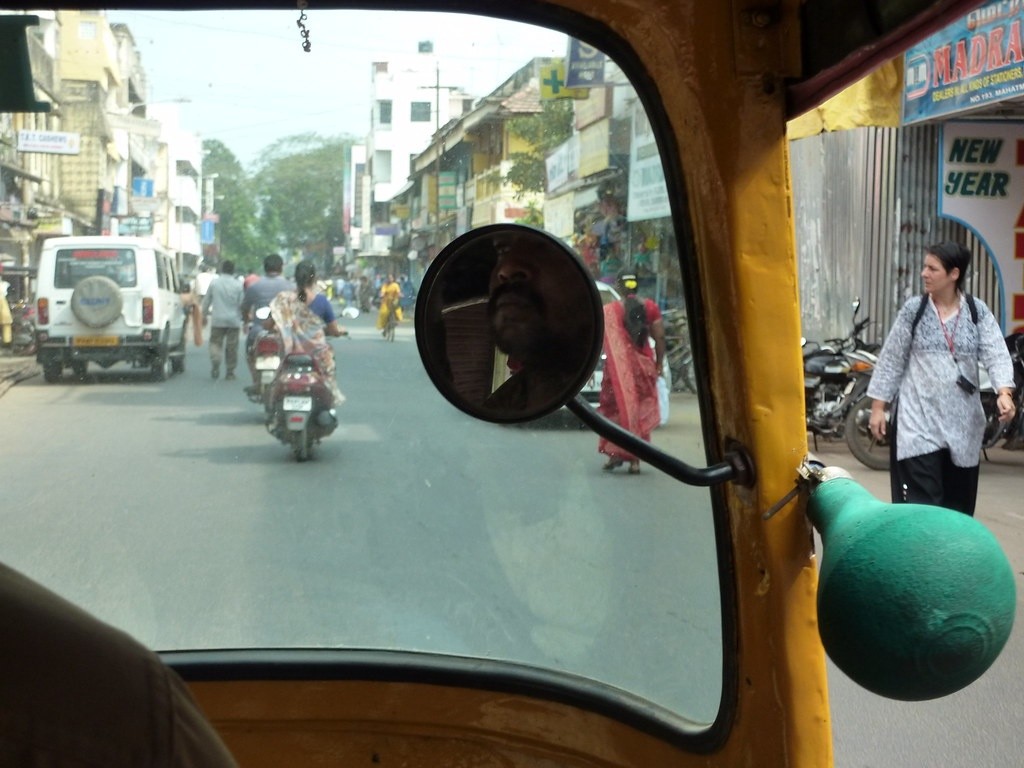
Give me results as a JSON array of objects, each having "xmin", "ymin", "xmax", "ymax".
[{"xmin": 35, "ymin": 236, "xmax": 193, "ymax": 382}]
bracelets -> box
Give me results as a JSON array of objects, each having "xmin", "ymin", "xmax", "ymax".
[{"xmin": 999, "ymin": 393, "xmax": 1011, "ymax": 396}]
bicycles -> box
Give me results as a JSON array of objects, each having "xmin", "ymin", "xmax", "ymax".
[{"xmin": 377, "ymin": 295, "xmax": 404, "ymax": 342}]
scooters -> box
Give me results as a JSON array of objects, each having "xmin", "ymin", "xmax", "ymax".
[{"xmin": 254, "ymin": 305, "xmax": 360, "ymax": 463}]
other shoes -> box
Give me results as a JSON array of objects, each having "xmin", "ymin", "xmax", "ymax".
[
  {"xmin": 226, "ymin": 373, "xmax": 236, "ymax": 380},
  {"xmin": 243, "ymin": 385, "xmax": 260, "ymax": 394},
  {"xmin": 602, "ymin": 459, "xmax": 623, "ymax": 470},
  {"xmin": 212, "ymin": 361, "xmax": 219, "ymax": 380},
  {"xmin": 629, "ymin": 466, "xmax": 640, "ymax": 474}
]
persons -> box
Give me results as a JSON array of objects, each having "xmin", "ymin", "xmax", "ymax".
[
  {"xmin": 592, "ymin": 267, "xmax": 666, "ymax": 474},
  {"xmin": 867, "ymin": 239, "xmax": 1017, "ymax": 518},
  {"xmin": 188, "ymin": 252, "xmax": 416, "ymax": 383},
  {"xmin": 470, "ymin": 233, "xmax": 601, "ymax": 413}
]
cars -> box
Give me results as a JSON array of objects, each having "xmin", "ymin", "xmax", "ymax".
[{"xmin": 576, "ymin": 281, "xmax": 646, "ymax": 416}]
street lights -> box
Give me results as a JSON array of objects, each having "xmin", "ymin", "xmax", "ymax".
[{"xmin": 125, "ymin": 99, "xmax": 191, "ymax": 215}]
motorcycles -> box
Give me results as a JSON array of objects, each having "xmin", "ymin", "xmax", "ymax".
[
  {"xmin": 243, "ymin": 315, "xmax": 284, "ymax": 412},
  {"xmin": 801, "ymin": 299, "xmax": 1024, "ymax": 474}
]
